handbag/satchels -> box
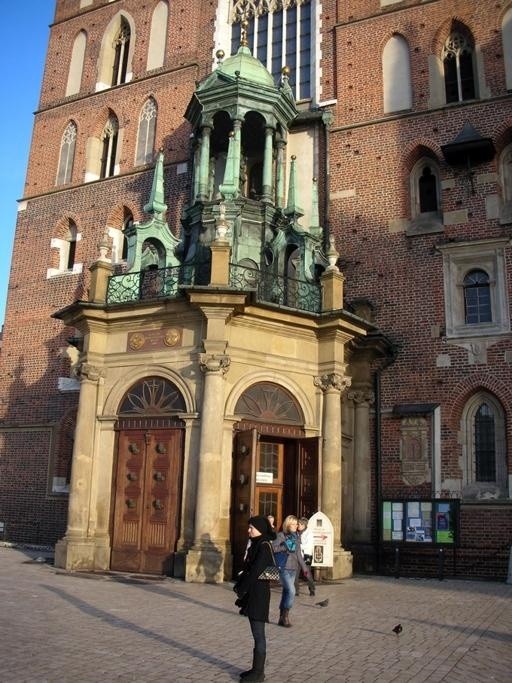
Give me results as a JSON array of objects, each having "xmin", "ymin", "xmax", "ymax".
[
  {"xmin": 257, "ymin": 551, "xmax": 289, "ymax": 584},
  {"xmin": 304, "ymin": 554, "xmax": 312, "ymax": 565}
]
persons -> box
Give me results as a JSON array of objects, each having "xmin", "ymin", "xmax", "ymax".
[
  {"xmin": 272, "ymin": 515, "xmax": 315, "ymax": 627},
  {"xmin": 233, "ymin": 514, "xmax": 280, "ymax": 683}
]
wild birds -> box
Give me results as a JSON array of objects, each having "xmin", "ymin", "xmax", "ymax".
[
  {"xmin": 392, "ymin": 624, "xmax": 403, "ymax": 636},
  {"xmin": 315, "ymin": 598, "xmax": 330, "ymax": 607}
]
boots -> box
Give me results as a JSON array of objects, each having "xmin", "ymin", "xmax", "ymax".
[
  {"xmin": 239, "ymin": 648, "xmax": 266, "ymax": 682},
  {"xmin": 278, "ymin": 608, "xmax": 292, "ymax": 627},
  {"xmin": 295, "ymin": 584, "xmax": 315, "ymax": 597}
]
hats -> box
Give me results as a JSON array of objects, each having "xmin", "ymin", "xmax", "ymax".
[{"xmin": 249, "ymin": 516, "xmax": 272, "ymax": 533}]
